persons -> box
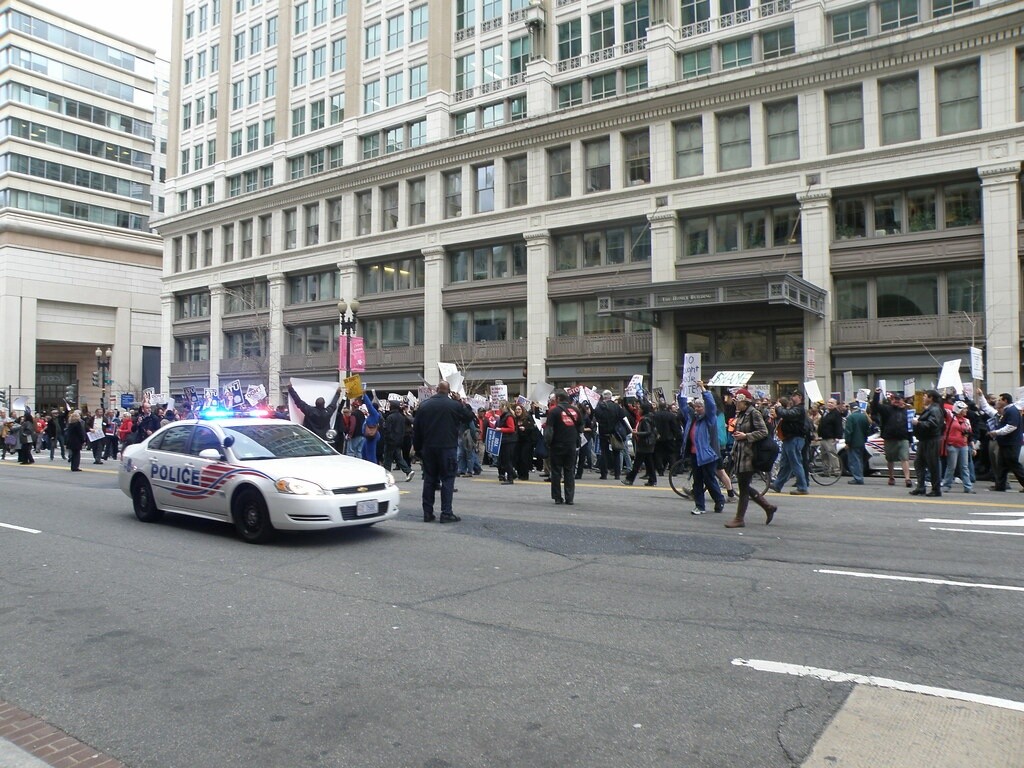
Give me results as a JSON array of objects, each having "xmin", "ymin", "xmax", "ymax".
[
  {"xmin": 131, "ymin": 401, "xmax": 290, "ymax": 443},
  {"xmin": 627, "ymin": 380, "xmax": 913, "ymax": 528},
  {"xmin": 0, "ymin": 408, "xmax": 133, "ymax": 472},
  {"xmin": 477, "ymin": 389, "xmax": 626, "ymax": 504},
  {"xmin": 287, "ymin": 381, "xmax": 475, "ymax": 523},
  {"xmin": 909, "ymin": 386, "xmax": 1024, "ymax": 497}
]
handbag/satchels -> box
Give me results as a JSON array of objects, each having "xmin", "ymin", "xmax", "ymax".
[
  {"xmin": 940, "ymin": 440, "xmax": 948, "ymax": 457},
  {"xmin": 535, "ymin": 439, "xmax": 548, "ymax": 458},
  {"xmin": 4, "ymin": 434, "xmax": 16, "ymax": 445},
  {"xmin": 365, "ymin": 425, "xmax": 377, "ymax": 439},
  {"xmin": 576, "ymin": 435, "xmax": 582, "ymax": 448},
  {"xmin": 610, "ymin": 434, "xmax": 625, "ymax": 450},
  {"xmin": 752, "ymin": 437, "xmax": 779, "ymax": 472}
]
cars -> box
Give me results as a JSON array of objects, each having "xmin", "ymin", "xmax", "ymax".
[
  {"xmin": 813, "ymin": 426, "xmax": 919, "ymax": 475},
  {"xmin": 117, "ymin": 405, "xmax": 402, "ymax": 545}
]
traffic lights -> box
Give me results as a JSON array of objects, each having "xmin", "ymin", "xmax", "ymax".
[
  {"xmin": 66, "ymin": 386, "xmax": 72, "ymax": 404},
  {"xmin": 0, "ymin": 389, "xmax": 7, "ymax": 403},
  {"xmin": 92, "ymin": 372, "xmax": 100, "ymax": 388},
  {"xmin": 99, "ymin": 397, "xmax": 104, "ymax": 408}
]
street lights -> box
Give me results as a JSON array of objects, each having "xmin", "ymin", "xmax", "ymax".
[
  {"xmin": 96, "ymin": 346, "xmax": 112, "ymax": 411},
  {"xmin": 337, "ymin": 297, "xmax": 360, "ymax": 412}
]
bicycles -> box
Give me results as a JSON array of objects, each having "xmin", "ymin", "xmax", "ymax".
[{"xmin": 758, "ymin": 442, "xmax": 844, "ymax": 486}]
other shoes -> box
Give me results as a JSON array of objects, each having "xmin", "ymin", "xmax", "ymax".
[
  {"xmin": 392, "ymin": 462, "xmax": 1024, "ymax": 529},
  {"xmin": 0, "ymin": 445, "xmax": 122, "ymax": 471}
]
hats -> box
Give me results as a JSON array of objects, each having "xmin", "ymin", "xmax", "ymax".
[
  {"xmin": 850, "ymin": 403, "xmax": 859, "ymax": 409},
  {"xmin": 478, "ymin": 408, "xmax": 486, "ymax": 412},
  {"xmin": 790, "ymin": 389, "xmax": 803, "ymax": 397},
  {"xmin": 893, "ymin": 392, "xmax": 904, "ymax": 399},
  {"xmin": 352, "ymin": 402, "xmax": 359, "ymax": 406},
  {"xmin": 732, "ymin": 389, "xmax": 752, "ymax": 402},
  {"xmin": 389, "ymin": 400, "xmax": 400, "ymax": 406}
]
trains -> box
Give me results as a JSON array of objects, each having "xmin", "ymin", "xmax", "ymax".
[{"xmin": 668, "ymin": 443, "xmax": 773, "ymax": 500}]
